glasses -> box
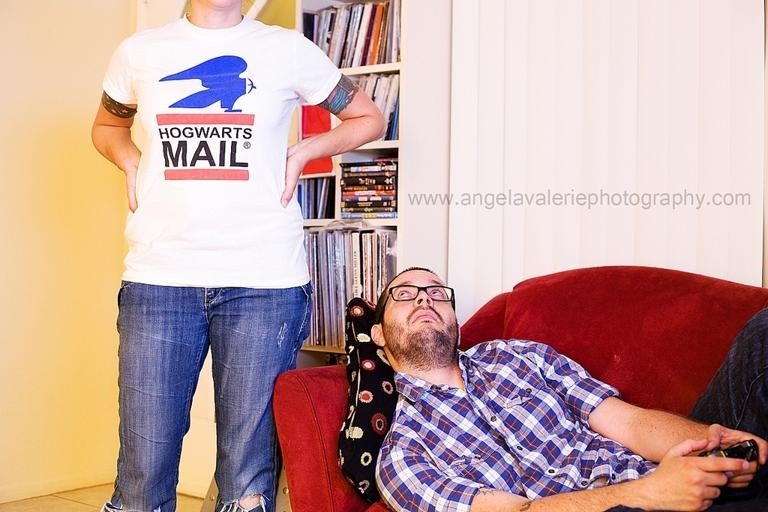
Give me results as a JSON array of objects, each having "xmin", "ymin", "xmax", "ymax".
[{"xmin": 377, "ymin": 285, "xmax": 455, "ymax": 323}]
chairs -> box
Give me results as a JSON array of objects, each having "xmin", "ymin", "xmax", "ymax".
[{"xmin": 273, "ymin": 265, "xmax": 768, "ymax": 511}]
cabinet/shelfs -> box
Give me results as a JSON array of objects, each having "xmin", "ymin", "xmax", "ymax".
[{"xmin": 295, "ymin": 0, "xmax": 405, "ymax": 356}]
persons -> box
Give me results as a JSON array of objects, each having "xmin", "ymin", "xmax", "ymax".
[
  {"xmin": 373, "ymin": 267, "xmax": 768, "ymax": 511},
  {"xmin": 90, "ymin": 2, "xmax": 388, "ymax": 512}
]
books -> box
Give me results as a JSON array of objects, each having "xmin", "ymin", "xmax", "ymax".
[{"xmin": 300, "ymin": 0, "xmax": 401, "ymax": 365}]
